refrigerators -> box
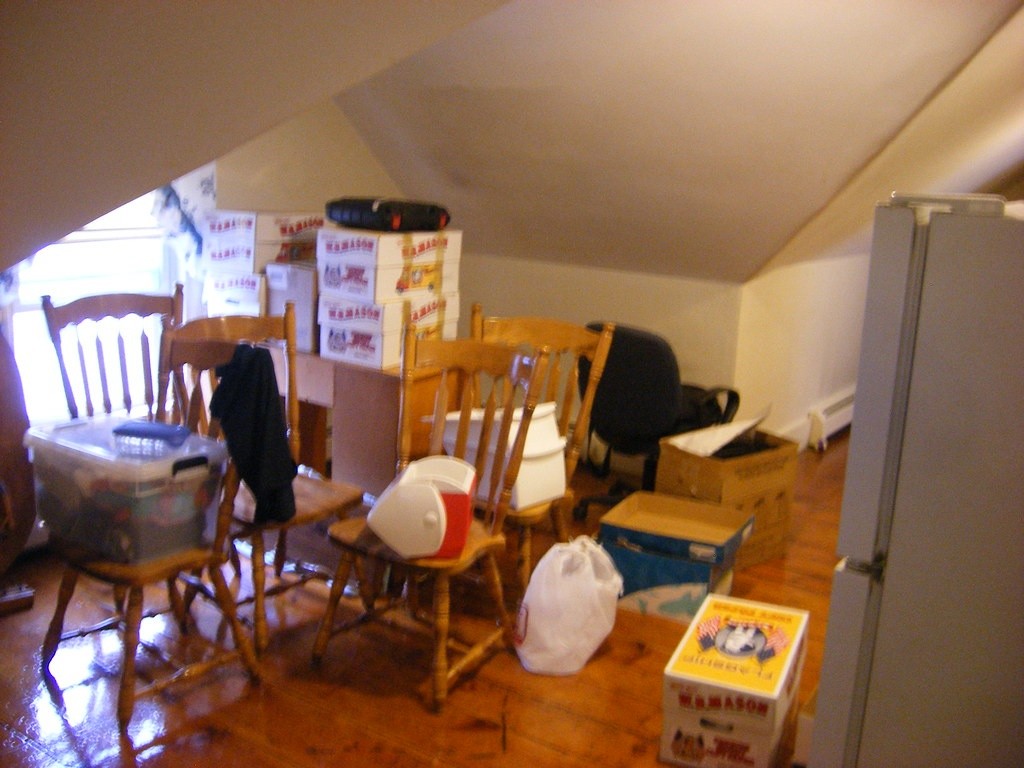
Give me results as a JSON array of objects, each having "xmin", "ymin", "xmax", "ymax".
[{"xmin": 802, "ymin": 190, "xmax": 1024, "ymax": 768}]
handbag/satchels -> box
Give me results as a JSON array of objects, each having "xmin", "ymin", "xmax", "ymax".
[{"xmin": 516, "ymin": 536, "xmax": 623, "ymax": 679}]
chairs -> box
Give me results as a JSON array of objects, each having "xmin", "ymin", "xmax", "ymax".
[
  {"xmin": 37, "ymin": 313, "xmax": 268, "ymax": 724},
  {"xmin": 313, "ymin": 320, "xmax": 519, "ymax": 709},
  {"xmin": 155, "ymin": 303, "xmax": 377, "ymax": 654},
  {"xmin": 43, "ymin": 284, "xmax": 244, "ymax": 581},
  {"xmin": 577, "ymin": 321, "xmax": 740, "ymax": 489},
  {"xmin": 395, "ymin": 306, "xmax": 614, "ymax": 595}
]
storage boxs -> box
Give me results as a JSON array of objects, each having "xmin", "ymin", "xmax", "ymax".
[
  {"xmin": 201, "ymin": 210, "xmax": 462, "ymax": 370},
  {"xmin": 659, "ymin": 592, "xmax": 810, "ymax": 768},
  {"xmin": 421, "ymin": 402, "xmax": 560, "ymax": 454},
  {"xmin": 655, "ymin": 426, "xmax": 799, "ymax": 572},
  {"xmin": 444, "ymin": 439, "xmax": 568, "ymax": 511},
  {"xmin": 23, "ymin": 413, "xmax": 228, "ymax": 565},
  {"xmin": 598, "ymin": 489, "xmax": 757, "ymax": 624}
]
